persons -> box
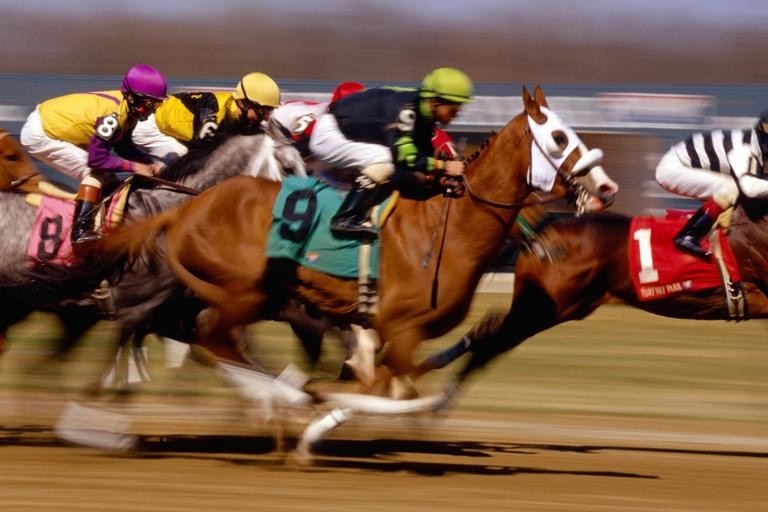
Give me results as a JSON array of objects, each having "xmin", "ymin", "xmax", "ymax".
[
  {"xmin": 18, "ymin": 64, "xmax": 168, "ymax": 246},
  {"xmin": 131, "ymin": 72, "xmax": 281, "ymax": 173},
  {"xmin": 307, "ymin": 67, "xmax": 475, "ymax": 240},
  {"xmin": 653, "ymin": 114, "xmax": 768, "ymax": 261},
  {"xmin": 270, "ymin": 80, "xmax": 369, "ymax": 144}
]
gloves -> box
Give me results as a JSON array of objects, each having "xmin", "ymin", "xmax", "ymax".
[{"xmin": 436, "ymin": 174, "xmax": 467, "ymax": 200}]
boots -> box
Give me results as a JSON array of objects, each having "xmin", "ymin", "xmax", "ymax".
[
  {"xmin": 328, "ymin": 170, "xmax": 383, "ymax": 240},
  {"xmin": 672, "ymin": 197, "xmax": 723, "ymax": 262},
  {"xmin": 71, "ymin": 184, "xmax": 105, "ymax": 241}
]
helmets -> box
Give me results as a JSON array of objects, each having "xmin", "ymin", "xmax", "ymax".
[
  {"xmin": 234, "ymin": 70, "xmax": 283, "ymax": 108},
  {"xmin": 328, "ymin": 81, "xmax": 368, "ymax": 105},
  {"xmin": 121, "ymin": 64, "xmax": 168, "ymax": 102},
  {"xmin": 418, "ymin": 67, "xmax": 479, "ymax": 103}
]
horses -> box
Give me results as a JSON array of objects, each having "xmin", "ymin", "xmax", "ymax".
[
  {"xmin": 405, "ymin": 197, "xmax": 767, "ymax": 415},
  {"xmin": 0, "ymin": 84, "xmax": 620, "ymax": 469}
]
